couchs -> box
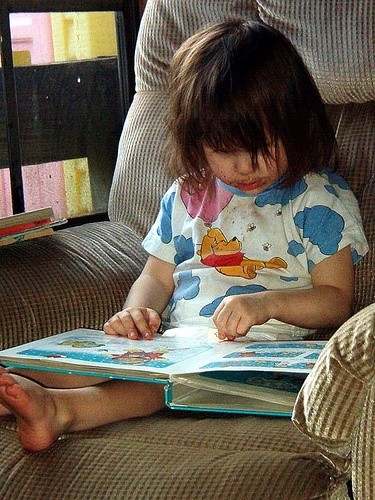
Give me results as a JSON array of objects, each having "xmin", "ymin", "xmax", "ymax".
[{"xmin": 0, "ymin": 0, "xmax": 375, "ymax": 500}]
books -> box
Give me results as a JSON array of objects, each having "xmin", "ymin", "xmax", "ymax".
[
  {"xmin": 0, "ymin": 329, "xmax": 331, "ymax": 415},
  {"xmin": 0, "ymin": 207, "xmax": 53, "ymax": 244}
]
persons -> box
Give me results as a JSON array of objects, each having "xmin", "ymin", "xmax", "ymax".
[{"xmin": 0, "ymin": 19, "xmax": 369, "ymax": 451}]
